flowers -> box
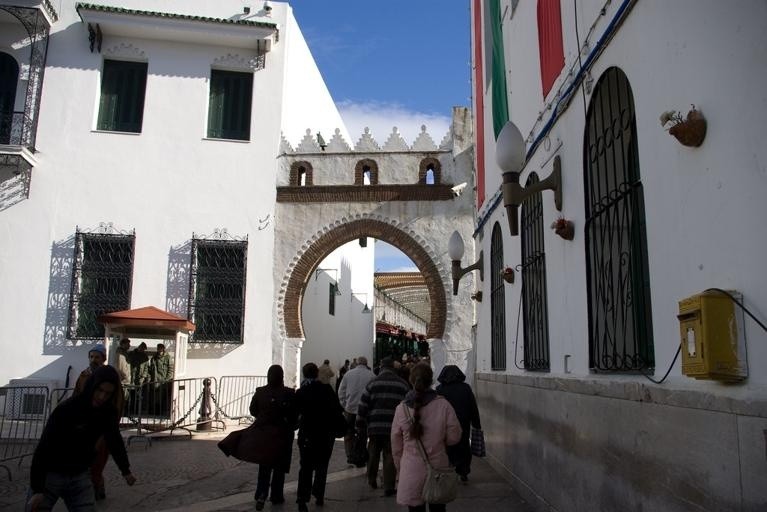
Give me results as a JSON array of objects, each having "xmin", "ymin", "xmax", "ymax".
[
  {"xmin": 659, "ymin": 104, "xmax": 703, "ymax": 136},
  {"xmin": 502, "ymin": 268, "xmax": 514, "ymax": 278},
  {"xmin": 550, "ymin": 216, "xmax": 567, "ymax": 231}
]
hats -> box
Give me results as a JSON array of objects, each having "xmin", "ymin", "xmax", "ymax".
[
  {"xmin": 88, "ymin": 344, "xmax": 107, "ymax": 362},
  {"xmin": 379, "ymin": 356, "xmax": 395, "ymax": 367}
]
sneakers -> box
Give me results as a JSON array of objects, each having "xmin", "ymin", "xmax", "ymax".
[
  {"xmin": 382, "ymin": 488, "xmax": 398, "ymax": 497},
  {"xmin": 254, "ymin": 490, "xmax": 268, "ymax": 509},
  {"xmin": 296, "ymin": 501, "xmax": 309, "ymax": 512}
]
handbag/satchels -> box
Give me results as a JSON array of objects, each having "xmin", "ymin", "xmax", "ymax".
[
  {"xmin": 334, "ymin": 412, "xmax": 351, "ymax": 439},
  {"xmin": 419, "ymin": 465, "xmax": 462, "ymax": 504},
  {"xmin": 470, "ymin": 429, "xmax": 487, "ymax": 457}
]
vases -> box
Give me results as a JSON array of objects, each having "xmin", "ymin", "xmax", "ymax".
[
  {"xmin": 559, "ymin": 221, "xmax": 574, "ymax": 241},
  {"xmin": 669, "ymin": 118, "xmax": 707, "ymax": 147},
  {"xmin": 503, "ymin": 272, "xmax": 514, "ymax": 283}
]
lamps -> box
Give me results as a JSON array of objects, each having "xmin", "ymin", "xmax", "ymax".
[
  {"xmin": 447, "ymin": 230, "xmax": 484, "ymax": 295},
  {"xmin": 494, "ymin": 120, "xmax": 562, "ymax": 236},
  {"xmin": 351, "ymin": 293, "xmax": 370, "ymax": 313},
  {"xmin": 316, "ymin": 269, "xmax": 341, "ymax": 296},
  {"xmin": 372, "ymin": 306, "xmax": 386, "ymax": 323}
]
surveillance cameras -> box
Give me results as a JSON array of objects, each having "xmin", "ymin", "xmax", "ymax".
[
  {"xmin": 263, "ymin": 1, "xmax": 273, "ymax": 14},
  {"xmin": 451, "ymin": 182, "xmax": 470, "ymax": 197}
]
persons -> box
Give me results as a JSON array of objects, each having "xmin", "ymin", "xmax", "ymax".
[
  {"xmin": 149, "ymin": 343, "xmax": 174, "ymax": 417},
  {"xmin": 127, "ymin": 341, "xmax": 150, "ymax": 417},
  {"xmin": 72, "ymin": 344, "xmax": 136, "ymax": 495},
  {"xmin": 215, "ymin": 342, "xmax": 486, "ymax": 512},
  {"xmin": 114, "ymin": 338, "xmax": 132, "ymax": 418},
  {"xmin": 23, "ymin": 364, "xmax": 123, "ymax": 512}
]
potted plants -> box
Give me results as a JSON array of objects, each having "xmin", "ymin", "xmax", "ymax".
[{"xmin": 471, "ymin": 291, "xmax": 481, "ymax": 301}]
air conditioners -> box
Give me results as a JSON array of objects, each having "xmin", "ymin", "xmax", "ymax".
[{"xmin": 5, "ymin": 379, "xmax": 59, "ymax": 421}]
